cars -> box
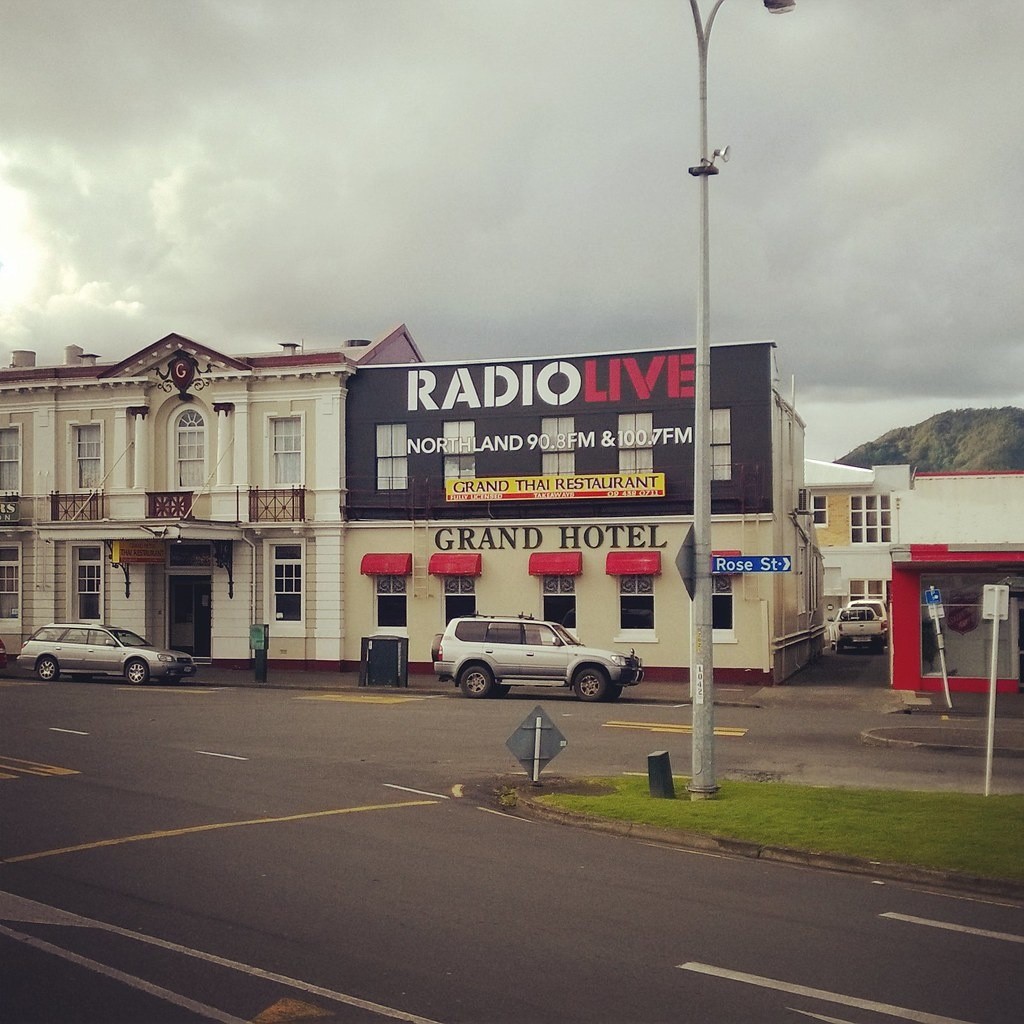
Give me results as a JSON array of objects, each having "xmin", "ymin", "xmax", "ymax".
[{"xmin": 14, "ymin": 620, "xmax": 199, "ymax": 686}]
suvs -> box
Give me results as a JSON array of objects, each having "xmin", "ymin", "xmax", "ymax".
[{"xmin": 431, "ymin": 612, "xmax": 645, "ymax": 702}]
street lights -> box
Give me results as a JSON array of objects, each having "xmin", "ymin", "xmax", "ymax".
[{"xmin": 689, "ymin": 1, "xmax": 795, "ymax": 803}]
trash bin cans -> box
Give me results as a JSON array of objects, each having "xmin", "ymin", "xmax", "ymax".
[{"xmin": 357, "ymin": 634, "xmax": 410, "ymax": 688}]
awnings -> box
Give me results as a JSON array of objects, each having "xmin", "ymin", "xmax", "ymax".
[
  {"xmin": 428, "ymin": 553, "xmax": 481, "ymax": 576},
  {"xmin": 528, "ymin": 552, "xmax": 582, "ymax": 576},
  {"xmin": 711, "ymin": 550, "xmax": 742, "ymax": 574},
  {"xmin": 605, "ymin": 551, "xmax": 661, "ymax": 575},
  {"xmin": 359, "ymin": 553, "xmax": 412, "ymax": 575}
]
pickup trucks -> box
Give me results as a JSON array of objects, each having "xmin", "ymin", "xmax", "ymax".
[{"xmin": 828, "ymin": 607, "xmax": 886, "ymax": 653}]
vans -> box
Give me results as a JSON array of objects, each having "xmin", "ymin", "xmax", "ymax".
[{"xmin": 846, "ymin": 599, "xmax": 888, "ymax": 646}]
